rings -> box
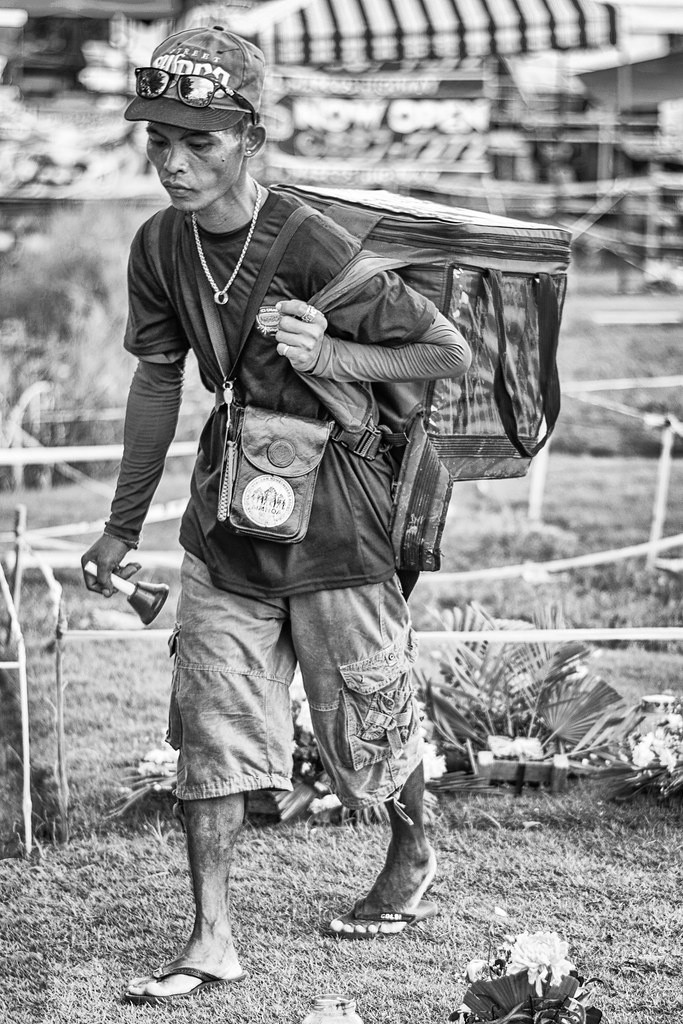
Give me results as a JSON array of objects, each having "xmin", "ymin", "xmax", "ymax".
[
  {"xmin": 302, "ymin": 305, "xmax": 317, "ymax": 322},
  {"xmin": 282, "ymin": 345, "xmax": 290, "ymax": 356}
]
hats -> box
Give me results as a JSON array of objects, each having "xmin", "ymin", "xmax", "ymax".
[{"xmin": 125, "ymin": 27, "xmax": 268, "ymax": 131}]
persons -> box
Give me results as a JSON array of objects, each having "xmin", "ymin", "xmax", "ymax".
[{"xmin": 81, "ymin": 28, "xmax": 472, "ymax": 1002}]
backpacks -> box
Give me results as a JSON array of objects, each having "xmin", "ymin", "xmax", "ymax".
[{"xmin": 269, "ymin": 178, "xmax": 573, "ymax": 482}]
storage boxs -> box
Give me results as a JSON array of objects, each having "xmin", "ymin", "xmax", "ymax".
[{"xmin": 267, "ymin": 183, "xmax": 570, "ymax": 483}]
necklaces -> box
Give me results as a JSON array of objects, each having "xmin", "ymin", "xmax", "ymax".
[{"xmin": 192, "ymin": 179, "xmax": 262, "ymax": 305}]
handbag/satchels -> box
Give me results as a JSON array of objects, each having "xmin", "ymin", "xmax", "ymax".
[{"xmin": 211, "ymin": 408, "xmax": 333, "ymax": 547}]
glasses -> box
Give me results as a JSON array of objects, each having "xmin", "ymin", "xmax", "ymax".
[{"xmin": 132, "ymin": 66, "xmax": 258, "ymax": 124}]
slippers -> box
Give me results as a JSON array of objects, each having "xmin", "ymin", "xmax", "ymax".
[
  {"xmin": 125, "ymin": 966, "xmax": 249, "ymax": 1004},
  {"xmin": 327, "ymin": 896, "xmax": 438, "ymax": 938}
]
